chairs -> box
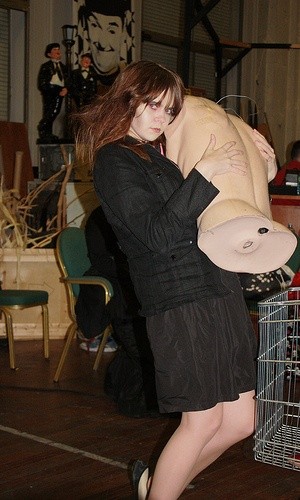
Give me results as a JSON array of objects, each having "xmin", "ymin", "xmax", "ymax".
[
  {"xmin": 53, "ymin": 227, "xmax": 118, "ymax": 384},
  {"xmin": 0, "ymin": 286, "xmax": 51, "ymax": 370}
]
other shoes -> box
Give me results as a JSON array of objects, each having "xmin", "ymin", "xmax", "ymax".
[{"xmin": 131, "ymin": 459, "xmax": 151, "ymax": 500}]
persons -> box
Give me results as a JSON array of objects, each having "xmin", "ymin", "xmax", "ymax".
[
  {"xmin": 93, "ymin": 57, "xmax": 278, "ymax": 500},
  {"xmin": 273, "ymin": 141, "xmax": 300, "ymax": 188},
  {"xmin": 36, "ymin": 41, "xmax": 96, "ymax": 140},
  {"xmin": 74, "ymin": 205, "xmax": 162, "ymax": 420}
]
